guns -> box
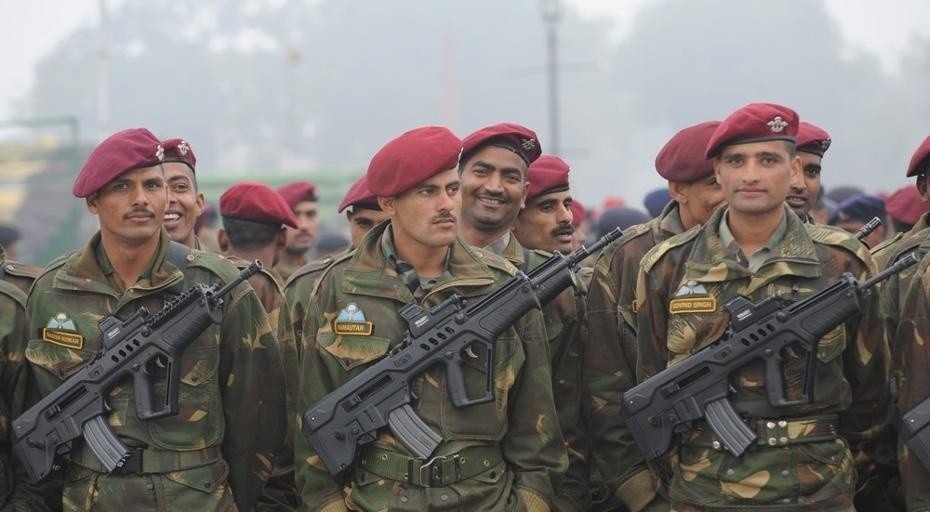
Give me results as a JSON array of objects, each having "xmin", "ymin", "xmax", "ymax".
[
  {"xmin": 852, "ymin": 215, "xmax": 882, "ymax": 244},
  {"xmin": 623, "ymin": 253, "xmax": 920, "ymax": 460},
  {"xmin": 302, "ymin": 227, "xmax": 625, "ymax": 478},
  {"xmin": 11, "ymin": 257, "xmax": 265, "ymax": 483}
]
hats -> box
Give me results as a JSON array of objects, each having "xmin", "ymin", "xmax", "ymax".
[
  {"xmin": 705, "ymin": 103, "xmax": 799, "ymax": 160},
  {"xmin": 163, "ymin": 139, "xmax": 196, "ymax": 171},
  {"xmin": 906, "ymin": 135, "xmax": 930, "ymax": 177},
  {"xmin": 338, "ymin": 176, "xmax": 381, "ymax": 214},
  {"xmin": 367, "ymin": 126, "xmax": 463, "ymax": 197},
  {"xmin": 221, "ymin": 183, "xmax": 299, "ymax": 229},
  {"xmin": 655, "ymin": 120, "xmax": 723, "ymax": 182},
  {"xmin": 73, "ymin": 128, "xmax": 164, "ymax": 198},
  {"xmin": 277, "ymin": 182, "xmax": 314, "ymax": 209},
  {"xmin": 525, "ymin": 156, "xmax": 569, "ymax": 199},
  {"xmin": 462, "ymin": 122, "xmax": 541, "ymax": 166},
  {"xmin": 886, "ymin": 186, "xmax": 925, "ymax": 225},
  {"xmin": 796, "ymin": 122, "xmax": 831, "ymax": 157}
]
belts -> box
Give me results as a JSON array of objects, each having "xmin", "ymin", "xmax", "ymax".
[
  {"xmin": 685, "ymin": 414, "xmax": 835, "ymax": 451},
  {"xmin": 68, "ymin": 441, "xmax": 221, "ymax": 473},
  {"xmin": 360, "ymin": 446, "xmax": 503, "ymax": 486}
]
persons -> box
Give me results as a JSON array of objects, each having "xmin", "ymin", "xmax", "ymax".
[
  {"xmin": 645, "ymin": 188, "xmax": 675, "ymax": 215},
  {"xmin": 159, "ymin": 138, "xmax": 300, "ymax": 411},
  {"xmin": 828, "ymin": 195, "xmax": 889, "ymax": 251},
  {"xmin": 302, "ymin": 126, "xmax": 569, "ymax": 511},
  {"xmin": 599, "ymin": 207, "xmax": 647, "ymax": 243},
  {"xmin": 25, "ymin": 128, "xmax": 292, "ymax": 511},
  {"xmin": 0, "ymin": 247, "xmax": 43, "ymax": 291},
  {"xmin": 788, "ymin": 124, "xmax": 831, "ymax": 226},
  {"xmin": 270, "ymin": 183, "xmax": 320, "ymax": 278},
  {"xmin": 588, "ymin": 122, "xmax": 727, "ymax": 512},
  {"xmin": 217, "ymin": 180, "xmax": 300, "ymax": 279},
  {"xmin": 867, "ymin": 136, "xmax": 930, "ymax": 268},
  {"xmin": 457, "ymin": 126, "xmax": 586, "ymax": 511},
  {"xmin": 879, "ymin": 224, "xmax": 929, "ymax": 510},
  {"xmin": 513, "ymin": 157, "xmax": 594, "ymax": 283},
  {"xmin": 0, "ymin": 280, "xmax": 44, "ymax": 511},
  {"xmin": 886, "ymin": 184, "xmax": 930, "ymax": 232},
  {"xmin": 282, "ymin": 170, "xmax": 396, "ymax": 341},
  {"xmin": 637, "ymin": 102, "xmax": 898, "ymax": 512}
]
